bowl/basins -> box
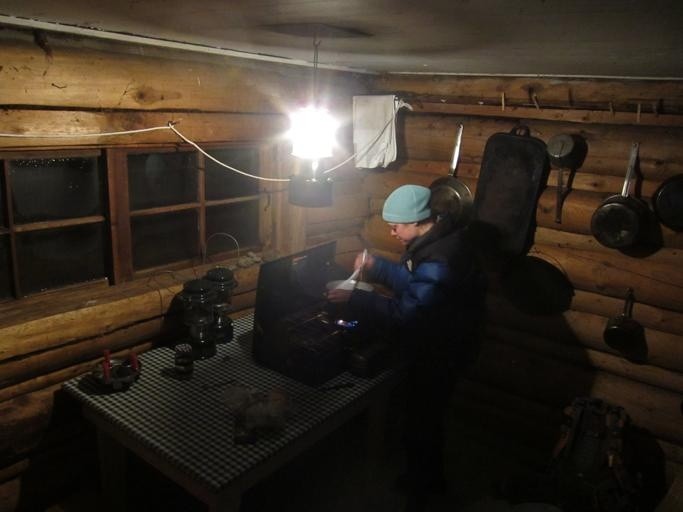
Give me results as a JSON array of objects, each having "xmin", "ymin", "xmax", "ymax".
[{"xmin": 90, "ymin": 357, "xmax": 141, "ymax": 391}]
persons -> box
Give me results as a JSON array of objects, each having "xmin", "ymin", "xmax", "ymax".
[{"xmin": 322, "ymin": 181, "xmax": 466, "ymax": 495}]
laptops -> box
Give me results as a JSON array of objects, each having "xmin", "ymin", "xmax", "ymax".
[{"xmin": 254, "ymin": 240, "xmax": 349, "ymax": 344}]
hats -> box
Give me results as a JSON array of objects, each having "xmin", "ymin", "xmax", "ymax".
[{"xmin": 381, "ymin": 183, "xmax": 432, "ymax": 224}]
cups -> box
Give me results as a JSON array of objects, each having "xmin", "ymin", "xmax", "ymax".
[{"xmin": 228, "ymin": 387, "xmax": 291, "ymax": 447}]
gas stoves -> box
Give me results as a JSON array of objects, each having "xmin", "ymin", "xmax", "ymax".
[{"xmin": 267, "ymin": 300, "xmax": 379, "ymax": 384}]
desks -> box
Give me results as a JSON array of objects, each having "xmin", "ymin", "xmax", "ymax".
[{"xmin": 70, "ymin": 305, "xmax": 395, "ymax": 512}]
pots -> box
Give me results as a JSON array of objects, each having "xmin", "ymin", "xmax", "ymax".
[
  {"xmin": 320, "ymin": 280, "xmax": 375, "ymax": 322},
  {"xmin": 420, "ymin": 123, "xmax": 473, "ymax": 233},
  {"xmin": 591, "ymin": 143, "xmax": 653, "ymax": 249},
  {"xmin": 540, "ymin": 132, "xmax": 585, "ymax": 225},
  {"xmin": 653, "ymin": 174, "xmax": 682, "ymax": 230},
  {"xmin": 602, "ymin": 287, "xmax": 643, "ymax": 349}
]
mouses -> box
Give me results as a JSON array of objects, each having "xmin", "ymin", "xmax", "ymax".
[{"xmin": 335, "ymin": 318, "xmax": 358, "ymax": 330}]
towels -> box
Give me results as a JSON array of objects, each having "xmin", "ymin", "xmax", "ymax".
[{"xmin": 353, "ymin": 92, "xmax": 407, "ymax": 170}]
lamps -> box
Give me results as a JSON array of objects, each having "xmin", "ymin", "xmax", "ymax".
[{"xmin": 283, "ymin": 35, "xmax": 339, "ymax": 212}]
